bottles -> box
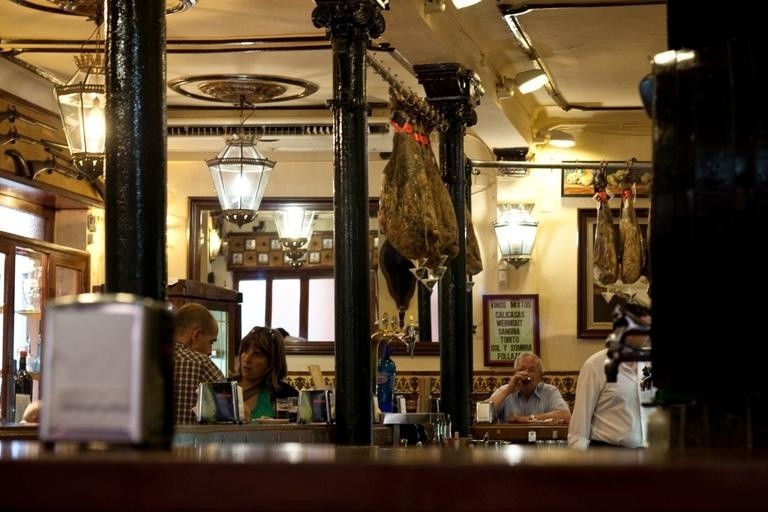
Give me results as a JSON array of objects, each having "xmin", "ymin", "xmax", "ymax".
[
  {"xmin": 377, "ymin": 344, "xmax": 395, "ymax": 411},
  {"xmin": 13, "ymin": 349, "xmax": 33, "ymax": 423},
  {"xmin": 22, "ymin": 334, "xmax": 41, "ymax": 401}
]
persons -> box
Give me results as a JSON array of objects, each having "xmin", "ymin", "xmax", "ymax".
[
  {"xmin": 567, "ymin": 299, "xmax": 656, "ymax": 451},
  {"xmin": 223, "ymin": 326, "xmax": 301, "ymax": 422},
  {"xmin": 483, "ymin": 352, "xmax": 571, "ymax": 428},
  {"xmin": 169, "ymin": 300, "xmax": 249, "ymax": 424}
]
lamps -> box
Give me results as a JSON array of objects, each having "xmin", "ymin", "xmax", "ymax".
[
  {"xmin": 51, "ymin": 0, "xmax": 109, "ymax": 184},
  {"xmin": 487, "ymin": 190, "xmax": 541, "ymax": 273},
  {"xmin": 495, "ymin": 68, "xmax": 548, "ymax": 101},
  {"xmin": 532, "ymin": 127, "xmax": 575, "ymax": 148},
  {"xmin": 423, "ymin": 0, "xmax": 482, "ymax": 14},
  {"xmin": 270, "ymin": 207, "xmax": 319, "ymax": 271},
  {"xmin": 193, "ymin": 79, "xmax": 293, "ymax": 228}
]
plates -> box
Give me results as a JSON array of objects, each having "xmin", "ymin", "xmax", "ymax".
[{"xmin": 255, "ymin": 418, "xmax": 289, "ymax": 423}]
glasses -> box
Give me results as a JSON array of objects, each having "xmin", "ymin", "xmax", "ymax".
[{"xmin": 252, "ymin": 326, "xmax": 277, "ymax": 341}]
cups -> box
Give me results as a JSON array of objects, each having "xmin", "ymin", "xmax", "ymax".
[
  {"xmin": 287, "ymin": 397, "xmax": 300, "ymax": 423},
  {"xmin": 520, "ymin": 372, "xmax": 536, "ymax": 385},
  {"xmin": 277, "ymin": 399, "xmax": 286, "ymax": 419},
  {"xmin": 19, "ymin": 268, "xmax": 39, "ymax": 312}
]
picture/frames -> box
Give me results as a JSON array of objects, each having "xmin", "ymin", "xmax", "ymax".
[
  {"xmin": 559, "ymin": 158, "xmax": 652, "ymax": 200},
  {"xmin": 574, "ymin": 203, "xmax": 651, "ymax": 342},
  {"xmin": 481, "ymin": 292, "xmax": 540, "ymax": 368},
  {"xmin": 231, "ymin": 237, "xmax": 333, "ymax": 267}
]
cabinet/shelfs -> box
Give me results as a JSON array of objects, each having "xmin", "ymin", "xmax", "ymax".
[{"xmin": 0, "ymin": 230, "xmax": 88, "ymax": 424}]
